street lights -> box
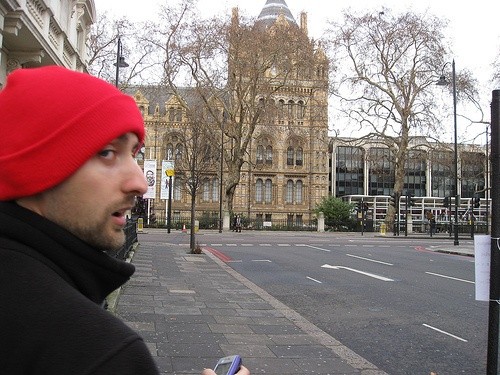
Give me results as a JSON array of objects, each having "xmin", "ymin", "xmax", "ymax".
[
  {"xmin": 113, "ymin": 37, "xmax": 132, "ymax": 88},
  {"xmin": 436, "ymin": 59, "xmax": 461, "ymax": 246}
]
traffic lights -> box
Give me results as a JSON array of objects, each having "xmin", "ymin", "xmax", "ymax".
[
  {"xmin": 474, "ymin": 197, "xmax": 481, "ymax": 208},
  {"xmin": 390, "ymin": 193, "xmax": 398, "ymax": 209},
  {"xmin": 355, "ymin": 200, "xmax": 361, "ymax": 213},
  {"xmin": 364, "ymin": 203, "xmax": 370, "ymax": 212},
  {"xmin": 442, "ymin": 197, "xmax": 450, "ymax": 208},
  {"xmin": 408, "ymin": 193, "xmax": 421, "ymax": 208}
]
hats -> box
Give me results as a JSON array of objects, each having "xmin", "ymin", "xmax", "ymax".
[{"xmin": 0, "ymin": 65, "xmax": 145, "ymax": 201}]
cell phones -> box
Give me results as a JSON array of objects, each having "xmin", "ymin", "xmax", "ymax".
[{"xmin": 214, "ymin": 355, "xmax": 241, "ymax": 375}]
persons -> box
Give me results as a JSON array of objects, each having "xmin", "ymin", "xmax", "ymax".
[
  {"xmin": 0, "ymin": 66, "xmax": 250, "ymax": 375},
  {"xmin": 232, "ymin": 214, "xmax": 241, "ymax": 232},
  {"xmin": 430, "ymin": 217, "xmax": 435, "ymax": 234}
]
